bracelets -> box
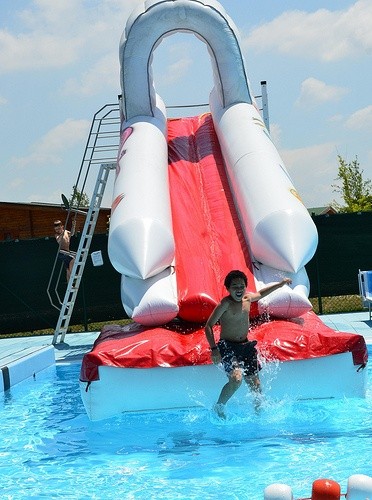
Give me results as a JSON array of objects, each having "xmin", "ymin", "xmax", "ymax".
[{"xmin": 211, "ymin": 346, "xmax": 218, "ymax": 351}]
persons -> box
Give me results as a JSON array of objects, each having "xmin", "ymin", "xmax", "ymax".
[
  {"xmin": 53, "ymin": 220, "xmax": 76, "ymax": 288},
  {"xmin": 204, "ymin": 270, "xmax": 293, "ymax": 422}
]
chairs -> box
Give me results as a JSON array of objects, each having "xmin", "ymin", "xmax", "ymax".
[{"xmin": 358, "ymin": 269, "xmax": 372, "ymax": 322}]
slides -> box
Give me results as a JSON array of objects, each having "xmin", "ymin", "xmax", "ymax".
[{"xmin": 107, "ymin": 113, "xmax": 318, "ymax": 326}]
